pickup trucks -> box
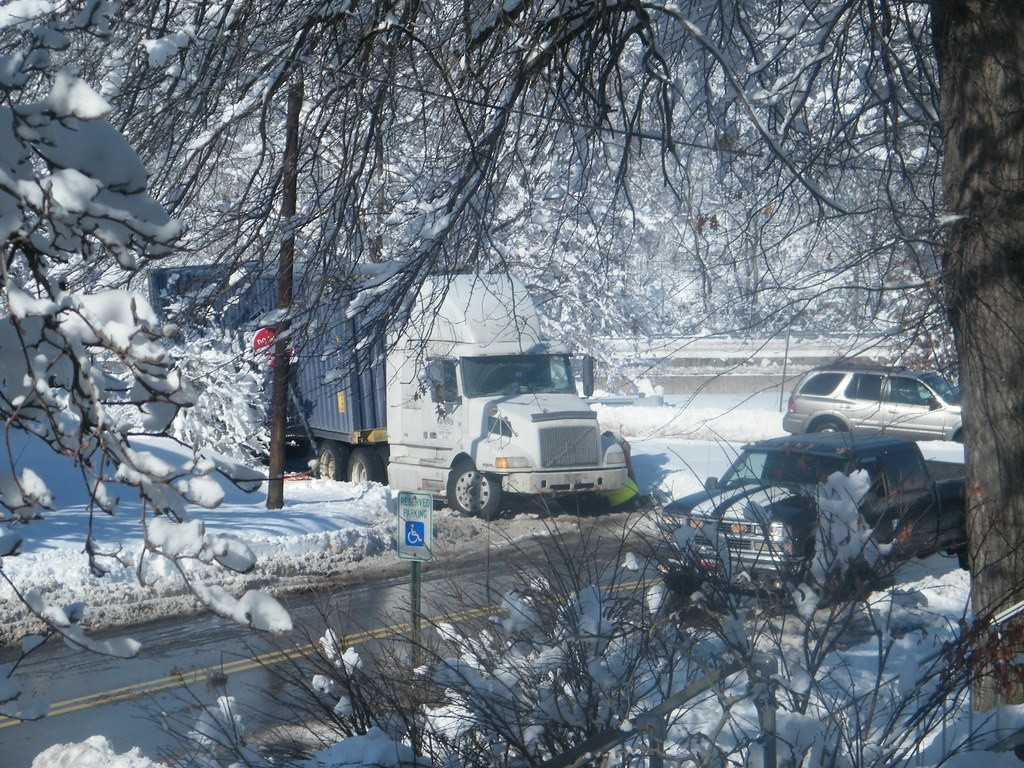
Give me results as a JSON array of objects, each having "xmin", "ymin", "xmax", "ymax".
[{"xmin": 661, "ymin": 430, "xmax": 972, "ymax": 605}]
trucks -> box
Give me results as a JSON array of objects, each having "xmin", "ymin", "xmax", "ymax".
[{"xmin": 146, "ymin": 257, "xmax": 625, "ymax": 515}]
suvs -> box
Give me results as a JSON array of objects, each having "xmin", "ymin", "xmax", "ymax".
[{"xmin": 781, "ymin": 362, "xmax": 965, "ymax": 447}]
